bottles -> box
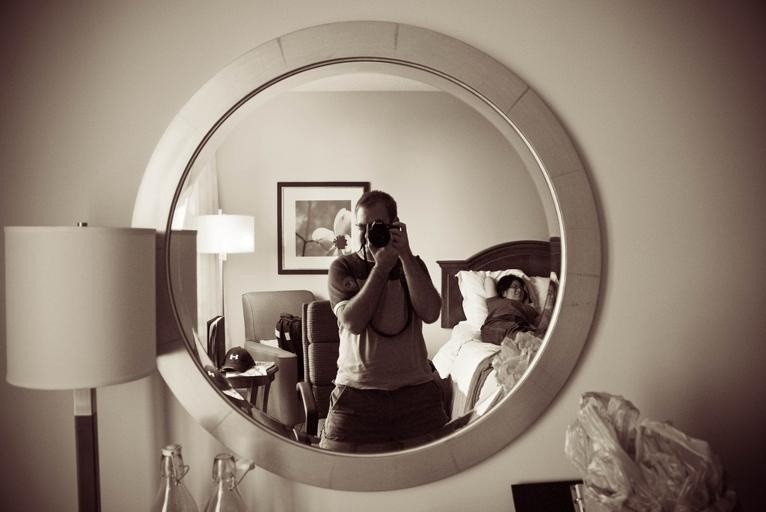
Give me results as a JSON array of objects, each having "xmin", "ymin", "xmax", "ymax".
[{"xmin": 151, "ymin": 443, "xmax": 247, "ymax": 511}]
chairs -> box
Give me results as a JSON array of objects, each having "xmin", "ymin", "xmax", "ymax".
[
  {"xmin": 294, "ymin": 298, "xmax": 350, "ymax": 449},
  {"xmin": 241, "ymin": 290, "xmax": 319, "ymax": 425}
]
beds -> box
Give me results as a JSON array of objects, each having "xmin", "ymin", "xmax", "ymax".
[{"xmin": 436, "ymin": 235, "xmax": 561, "ymax": 420}]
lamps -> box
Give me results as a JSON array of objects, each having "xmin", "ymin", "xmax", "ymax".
[
  {"xmin": 3, "ymin": 221, "xmax": 158, "ymax": 512},
  {"xmin": 195, "ymin": 207, "xmax": 255, "ymax": 351}
]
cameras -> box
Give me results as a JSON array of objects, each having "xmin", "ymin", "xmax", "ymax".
[{"xmin": 366, "ymin": 222, "xmax": 393, "ymax": 245}]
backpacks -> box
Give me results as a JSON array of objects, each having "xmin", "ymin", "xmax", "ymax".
[{"xmin": 274, "ymin": 312, "xmax": 304, "ymax": 382}]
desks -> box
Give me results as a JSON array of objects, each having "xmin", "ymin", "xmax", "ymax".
[{"xmin": 221, "ymin": 362, "xmax": 280, "ymax": 414}]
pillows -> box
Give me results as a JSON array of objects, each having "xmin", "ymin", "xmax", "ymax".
[
  {"xmin": 529, "ymin": 276, "xmax": 550, "ymax": 314},
  {"xmin": 455, "ymin": 267, "xmax": 531, "ymax": 331}
]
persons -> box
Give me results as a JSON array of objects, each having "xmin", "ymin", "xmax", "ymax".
[
  {"xmin": 317, "ymin": 187, "xmax": 452, "ymax": 454},
  {"xmin": 479, "ymin": 273, "xmax": 542, "ymax": 346}
]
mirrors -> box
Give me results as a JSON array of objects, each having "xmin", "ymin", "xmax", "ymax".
[{"xmin": 133, "ymin": 20, "xmax": 602, "ymax": 491}]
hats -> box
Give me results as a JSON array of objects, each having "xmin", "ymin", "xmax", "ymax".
[{"xmin": 221, "ymin": 346, "xmax": 257, "ymax": 374}]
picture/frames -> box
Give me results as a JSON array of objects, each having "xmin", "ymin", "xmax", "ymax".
[{"xmin": 277, "ymin": 181, "xmax": 372, "ymax": 275}]
